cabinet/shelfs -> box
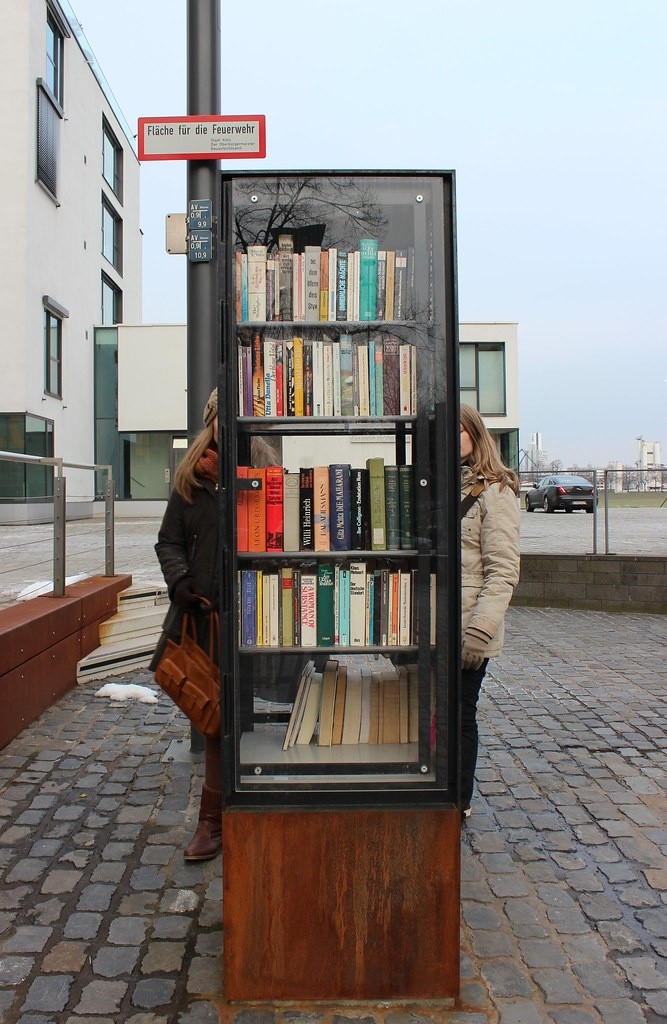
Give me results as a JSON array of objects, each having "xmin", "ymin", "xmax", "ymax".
[{"xmin": 219, "ymin": 169, "xmax": 461, "ymax": 1001}]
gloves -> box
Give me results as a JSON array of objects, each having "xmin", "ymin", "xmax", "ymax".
[
  {"xmin": 170, "ymin": 574, "xmax": 219, "ymax": 617},
  {"xmin": 461, "ymin": 633, "xmax": 487, "ymax": 670}
]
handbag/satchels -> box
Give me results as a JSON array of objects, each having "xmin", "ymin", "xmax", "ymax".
[{"xmin": 154, "ymin": 596, "xmax": 223, "ymax": 737}]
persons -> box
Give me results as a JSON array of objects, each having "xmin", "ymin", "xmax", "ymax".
[
  {"xmin": 149, "ymin": 388, "xmax": 223, "ymax": 859},
  {"xmin": 460, "ymin": 402, "xmax": 522, "ymax": 817}
]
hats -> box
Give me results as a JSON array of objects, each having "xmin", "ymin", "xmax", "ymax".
[{"xmin": 203, "ymin": 387, "xmax": 218, "ymax": 427}]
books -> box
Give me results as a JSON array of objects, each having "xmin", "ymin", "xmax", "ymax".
[
  {"xmin": 236, "ymin": 563, "xmax": 437, "ymax": 647},
  {"xmin": 236, "ymin": 234, "xmax": 414, "ymax": 322},
  {"xmin": 238, "ymin": 332, "xmax": 418, "ymax": 416},
  {"xmin": 237, "ymin": 457, "xmax": 414, "ymax": 551},
  {"xmin": 283, "ymin": 660, "xmax": 435, "ymax": 747}
]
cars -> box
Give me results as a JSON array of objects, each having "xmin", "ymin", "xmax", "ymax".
[{"xmin": 525, "ymin": 475, "xmax": 599, "ymax": 514}]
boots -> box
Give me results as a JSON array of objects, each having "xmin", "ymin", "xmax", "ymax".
[{"xmin": 183, "ymin": 783, "xmax": 223, "ymax": 860}]
